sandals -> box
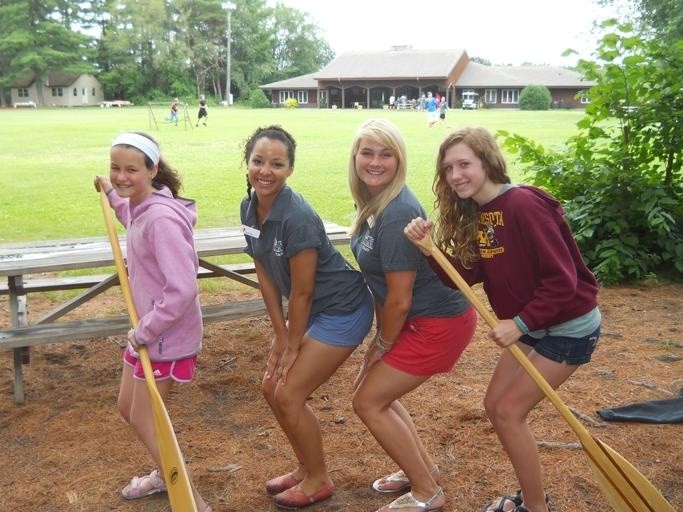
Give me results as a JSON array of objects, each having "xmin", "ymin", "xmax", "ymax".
[
  {"xmin": 122, "ymin": 468, "xmax": 171, "ymax": 501},
  {"xmin": 373, "ymin": 486, "xmax": 446, "ymax": 512},
  {"xmin": 370, "ymin": 464, "xmax": 442, "ymax": 495}
]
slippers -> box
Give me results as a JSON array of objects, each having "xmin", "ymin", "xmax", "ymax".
[
  {"xmin": 483, "ymin": 489, "xmax": 549, "ymax": 512},
  {"xmin": 508, "ymin": 505, "xmax": 553, "ymax": 512}
]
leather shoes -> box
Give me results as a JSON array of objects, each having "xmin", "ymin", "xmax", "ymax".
[
  {"xmin": 273, "ymin": 477, "xmax": 336, "ymax": 510},
  {"xmin": 265, "ymin": 473, "xmax": 303, "ymax": 495}
]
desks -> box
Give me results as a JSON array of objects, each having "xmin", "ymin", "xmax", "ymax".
[{"xmin": 0, "ymin": 220, "xmax": 356, "ymax": 400}]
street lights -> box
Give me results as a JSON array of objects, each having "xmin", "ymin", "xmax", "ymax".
[{"xmin": 221, "ymin": 0, "xmax": 236, "ymax": 105}]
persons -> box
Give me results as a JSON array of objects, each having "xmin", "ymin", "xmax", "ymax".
[
  {"xmin": 162, "ymin": 92, "xmax": 450, "ymax": 130},
  {"xmin": 405, "ymin": 126, "xmax": 603, "ymax": 512},
  {"xmin": 238, "ymin": 124, "xmax": 374, "ymax": 508},
  {"xmin": 348, "ymin": 118, "xmax": 477, "ymax": 511},
  {"xmin": 94, "ymin": 132, "xmax": 212, "ymax": 512}
]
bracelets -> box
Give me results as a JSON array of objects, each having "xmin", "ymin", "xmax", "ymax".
[
  {"xmin": 511, "ymin": 317, "xmax": 527, "ymax": 334},
  {"xmin": 376, "ymin": 330, "xmax": 394, "ymax": 353}
]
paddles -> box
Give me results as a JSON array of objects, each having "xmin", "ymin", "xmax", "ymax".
[
  {"xmin": 413, "ymin": 229, "xmax": 673, "ymax": 511},
  {"xmin": 97, "ymin": 183, "xmax": 196, "ymax": 512}
]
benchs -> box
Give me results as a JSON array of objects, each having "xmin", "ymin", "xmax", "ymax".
[
  {"xmin": 0, "ymin": 295, "xmax": 291, "ymax": 406},
  {"xmin": 0, "ymin": 260, "xmax": 260, "ymax": 358}
]
